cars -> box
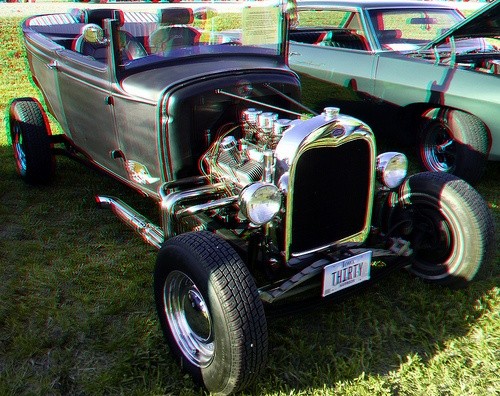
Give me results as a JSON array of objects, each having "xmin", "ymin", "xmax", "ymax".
[
  {"xmin": 9, "ymin": 7, "xmax": 494, "ymax": 396},
  {"xmin": 278, "ymin": 2, "xmax": 500, "ymax": 178}
]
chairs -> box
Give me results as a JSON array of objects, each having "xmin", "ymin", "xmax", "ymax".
[
  {"xmin": 320, "ymin": 29, "xmax": 406, "ymax": 51},
  {"xmin": 75, "ymin": 8, "xmax": 148, "ymax": 64},
  {"xmin": 149, "ymin": 7, "xmax": 197, "ymax": 53}
]
